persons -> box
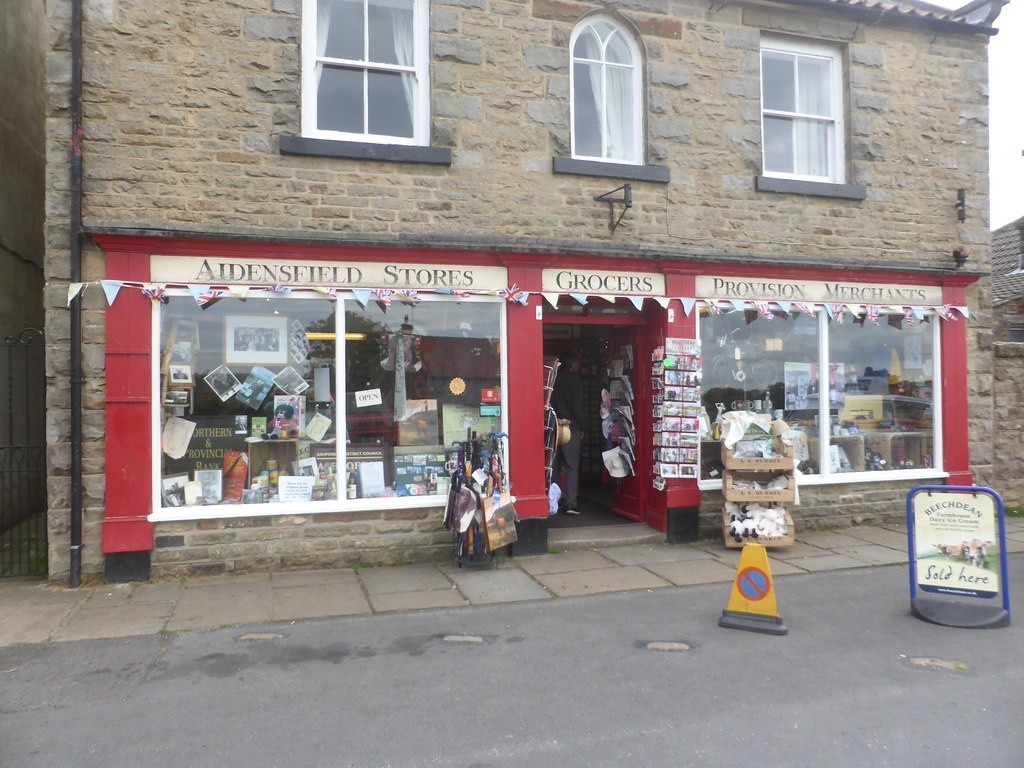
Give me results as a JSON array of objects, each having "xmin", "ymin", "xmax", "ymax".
[
  {"xmin": 244, "ymin": 378, "xmax": 265, "ymax": 404},
  {"xmin": 210, "ymin": 368, "xmax": 236, "ymax": 396},
  {"xmin": 545, "ymin": 352, "xmax": 585, "ymax": 514},
  {"xmin": 235, "ymin": 328, "xmax": 278, "ymax": 351}
]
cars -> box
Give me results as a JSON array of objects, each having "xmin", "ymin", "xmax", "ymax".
[
  {"xmin": 806, "ymin": 375, "xmax": 930, "ymax": 424},
  {"xmin": 781, "ymin": 392, "xmax": 854, "ymax": 437}
]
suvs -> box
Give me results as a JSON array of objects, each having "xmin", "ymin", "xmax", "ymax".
[{"xmin": 328, "ymin": 392, "xmax": 404, "ymax": 454}]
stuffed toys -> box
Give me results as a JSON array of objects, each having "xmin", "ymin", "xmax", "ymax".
[{"xmin": 262, "ymin": 404, "xmax": 297, "ymax": 440}]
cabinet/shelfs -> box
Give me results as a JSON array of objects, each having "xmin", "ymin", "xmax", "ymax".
[
  {"xmin": 811, "ymin": 433, "xmax": 928, "ymax": 473},
  {"xmin": 718, "ymin": 417, "xmax": 796, "ymax": 548}
]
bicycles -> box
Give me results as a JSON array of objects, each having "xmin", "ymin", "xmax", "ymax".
[{"xmin": 711, "ymin": 330, "xmax": 778, "ymax": 388}]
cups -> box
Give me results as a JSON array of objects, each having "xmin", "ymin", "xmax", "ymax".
[
  {"xmin": 731, "ymin": 400, "xmax": 743, "ymax": 411},
  {"xmin": 774, "ymin": 409, "xmax": 784, "ymax": 419},
  {"xmin": 833, "ymin": 424, "xmax": 859, "ymax": 436},
  {"xmin": 744, "ymin": 400, "xmax": 753, "ymax": 409},
  {"xmin": 753, "ymin": 400, "xmax": 762, "ymax": 409}
]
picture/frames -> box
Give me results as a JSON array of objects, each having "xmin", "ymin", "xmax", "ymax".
[
  {"xmin": 223, "ymin": 311, "xmax": 291, "ymax": 368},
  {"xmin": 168, "ymin": 362, "xmax": 196, "ymax": 387}
]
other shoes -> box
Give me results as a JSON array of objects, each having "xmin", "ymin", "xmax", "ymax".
[{"xmin": 566, "ymin": 507, "xmax": 580, "ymax": 514}]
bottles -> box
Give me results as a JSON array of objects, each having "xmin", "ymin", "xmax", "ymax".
[
  {"xmin": 349, "ymin": 471, "xmax": 356, "ymax": 499},
  {"xmin": 281, "ymin": 424, "xmax": 290, "ymax": 439}
]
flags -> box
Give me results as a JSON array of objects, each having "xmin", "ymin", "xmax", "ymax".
[{"xmin": 65, "ymin": 280, "xmax": 970, "ymax": 331}]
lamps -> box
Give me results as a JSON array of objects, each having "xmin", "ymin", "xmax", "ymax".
[{"xmin": 305, "ymin": 333, "xmax": 369, "ymax": 341}]
